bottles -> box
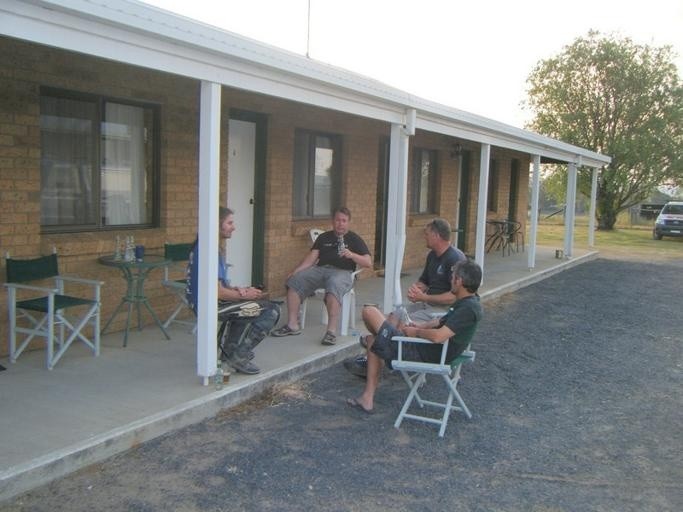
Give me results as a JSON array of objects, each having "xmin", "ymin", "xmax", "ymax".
[
  {"xmin": 336, "ymin": 235, "xmax": 344, "ymax": 255},
  {"xmin": 113, "ymin": 233, "xmax": 135, "ymax": 262},
  {"xmin": 401, "ymin": 311, "xmax": 413, "ymax": 328},
  {"xmin": 213, "ymin": 359, "xmax": 224, "ymax": 390}
]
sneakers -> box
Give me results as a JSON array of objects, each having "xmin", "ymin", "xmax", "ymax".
[{"xmin": 224, "ymin": 358, "xmax": 260, "ymax": 375}]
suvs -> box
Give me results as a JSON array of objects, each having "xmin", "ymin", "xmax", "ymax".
[{"xmin": 653, "ymin": 199, "xmax": 683, "ymax": 240}]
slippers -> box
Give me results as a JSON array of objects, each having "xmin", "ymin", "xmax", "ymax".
[
  {"xmin": 271, "ymin": 325, "xmax": 301, "ymax": 337},
  {"xmin": 321, "ymin": 329, "xmax": 337, "ymax": 346},
  {"xmin": 347, "ymin": 397, "xmax": 376, "ymax": 414}
]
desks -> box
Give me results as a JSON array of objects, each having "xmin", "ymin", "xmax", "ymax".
[{"xmin": 95, "ymin": 251, "xmax": 175, "ymax": 348}]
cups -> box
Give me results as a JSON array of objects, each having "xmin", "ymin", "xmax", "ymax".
[
  {"xmin": 134, "ymin": 245, "xmax": 144, "ymax": 263},
  {"xmin": 222, "ymin": 372, "xmax": 230, "ymax": 384}
]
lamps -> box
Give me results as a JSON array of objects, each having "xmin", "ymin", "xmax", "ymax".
[{"xmin": 399, "ymin": 108, "xmax": 417, "ymax": 136}]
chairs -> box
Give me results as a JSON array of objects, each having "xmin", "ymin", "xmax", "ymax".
[
  {"xmin": 388, "ymin": 312, "xmax": 483, "ymax": 438},
  {"xmin": 193, "ymin": 298, "xmax": 287, "ymax": 371},
  {"xmin": 3, "ymin": 248, "xmax": 104, "ymax": 373},
  {"xmin": 162, "ymin": 240, "xmax": 197, "ymax": 337},
  {"xmin": 296, "ymin": 228, "xmax": 358, "ymax": 337},
  {"xmin": 485, "ymin": 218, "xmax": 525, "ymax": 256},
  {"xmin": 385, "ymin": 292, "xmax": 479, "ymax": 363}
]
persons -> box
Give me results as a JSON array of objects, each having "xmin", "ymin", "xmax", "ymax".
[
  {"xmin": 346, "ymin": 262, "xmax": 482, "ymax": 415},
  {"xmin": 344, "ymin": 219, "xmax": 470, "ymax": 380},
  {"xmin": 272, "ymin": 207, "xmax": 371, "ymax": 345},
  {"xmin": 186, "ymin": 207, "xmax": 281, "ymax": 374}
]
